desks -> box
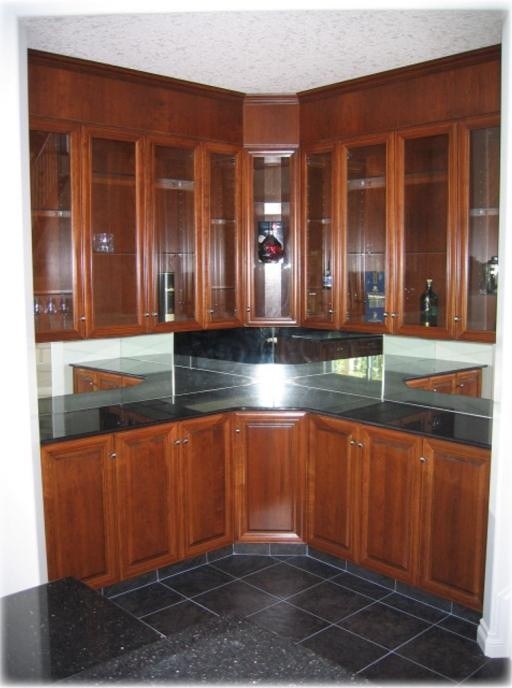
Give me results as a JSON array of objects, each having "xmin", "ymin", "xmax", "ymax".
[
  {"xmin": 57, "ymin": 610, "xmax": 373, "ymax": 686},
  {"xmin": 0, "ymin": 577, "xmax": 166, "ymax": 683}
]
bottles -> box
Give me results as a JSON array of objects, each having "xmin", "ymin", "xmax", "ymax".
[
  {"xmin": 158, "ymin": 271, "xmax": 175, "ymax": 323},
  {"xmin": 34, "ymin": 296, "xmax": 69, "ymax": 331},
  {"xmin": 322, "ymin": 258, "xmax": 331, "ymax": 288},
  {"xmin": 420, "ymin": 278, "xmax": 439, "ymax": 325}
]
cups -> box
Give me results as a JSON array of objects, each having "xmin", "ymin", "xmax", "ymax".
[{"xmin": 92, "ymin": 233, "xmax": 114, "ymax": 252}]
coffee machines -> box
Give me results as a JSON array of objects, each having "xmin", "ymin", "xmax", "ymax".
[{"xmin": 482, "ymin": 254, "xmax": 497, "ymax": 295}]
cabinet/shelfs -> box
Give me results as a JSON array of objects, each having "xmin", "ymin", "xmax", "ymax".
[
  {"xmin": 27, "ymin": 114, "xmax": 87, "ymax": 342},
  {"xmin": 304, "ymin": 411, "xmax": 424, "ymax": 587},
  {"xmin": 276, "ymin": 332, "xmax": 384, "ymax": 363},
  {"xmin": 116, "ymin": 412, "xmax": 236, "ymax": 582},
  {"xmin": 300, "ymin": 140, "xmax": 338, "ymax": 325},
  {"xmin": 418, "ymin": 433, "xmax": 491, "ymax": 614},
  {"xmin": 40, "ymin": 432, "xmax": 121, "ymax": 592},
  {"xmin": 200, "ymin": 137, "xmax": 248, "ymax": 328},
  {"xmin": 403, "ymin": 372, "xmax": 454, "ymax": 396},
  {"xmin": 241, "ymin": 144, "xmax": 300, "ymax": 330},
  {"xmin": 230, "ymin": 407, "xmax": 308, "ymax": 547},
  {"xmin": 340, "ymin": 128, "xmax": 459, "ymax": 331},
  {"xmin": 456, "ymin": 122, "xmax": 501, "ymax": 338},
  {"xmin": 79, "ymin": 124, "xmax": 204, "ymax": 338},
  {"xmin": 96, "ymin": 372, "xmax": 146, "ymax": 392},
  {"xmin": 72, "ymin": 365, "xmax": 97, "ymax": 394},
  {"xmin": 453, "ymin": 368, "xmax": 482, "ymax": 399}
]
